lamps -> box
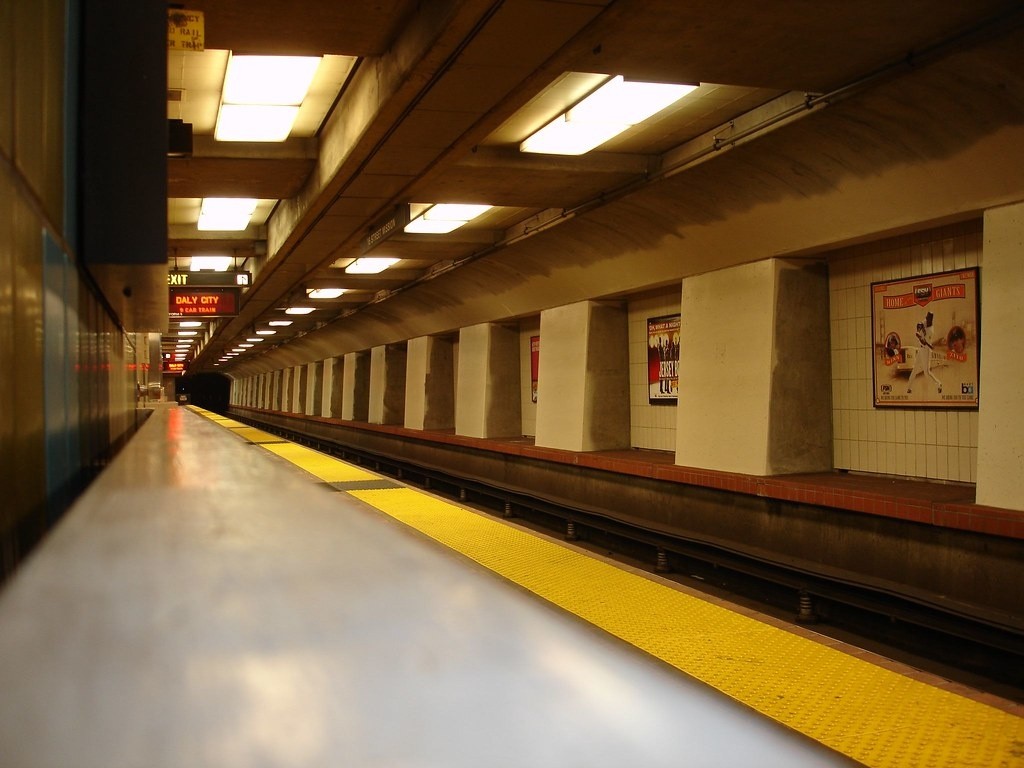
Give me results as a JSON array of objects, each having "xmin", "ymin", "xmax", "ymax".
[
  {"xmin": 196, "ymin": 198, "xmax": 261, "ymax": 232},
  {"xmin": 402, "ymin": 203, "xmax": 496, "ymax": 233},
  {"xmin": 213, "ymin": 37, "xmax": 325, "ymax": 142},
  {"xmin": 175, "ymin": 256, "xmax": 232, "ymax": 362},
  {"xmin": 344, "ymin": 257, "xmax": 402, "ymax": 275},
  {"xmin": 217, "ymin": 307, "xmax": 318, "ymax": 362},
  {"xmin": 306, "ymin": 288, "xmax": 349, "ymax": 299},
  {"xmin": 517, "ymin": 72, "xmax": 701, "ymax": 156}
]
